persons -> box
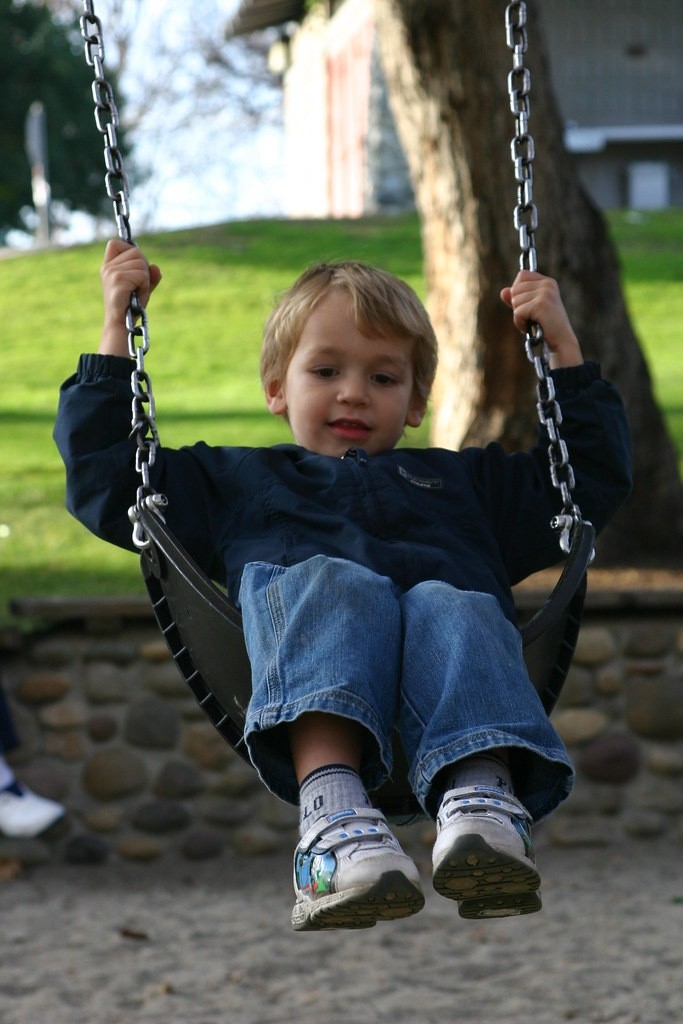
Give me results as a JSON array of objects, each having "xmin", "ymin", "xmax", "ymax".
[
  {"xmin": 53, "ymin": 239, "xmax": 633, "ymax": 933},
  {"xmin": 0, "ymin": 687, "xmax": 67, "ymax": 840}
]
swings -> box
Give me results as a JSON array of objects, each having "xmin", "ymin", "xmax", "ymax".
[{"xmin": 79, "ymin": 0, "xmax": 598, "ymax": 822}]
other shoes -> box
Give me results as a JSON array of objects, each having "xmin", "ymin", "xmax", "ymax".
[{"xmin": 0, "ymin": 770, "xmax": 67, "ymax": 840}]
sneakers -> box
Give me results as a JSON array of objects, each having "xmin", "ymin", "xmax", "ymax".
[
  {"xmin": 290, "ymin": 808, "xmax": 424, "ymax": 931},
  {"xmin": 432, "ymin": 785, "xmax": 542, "ymax": 920}
]
